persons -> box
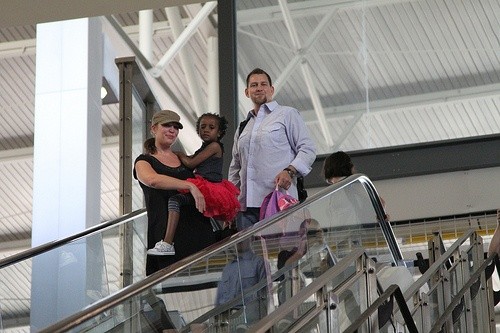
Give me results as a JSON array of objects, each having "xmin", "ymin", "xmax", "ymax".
[
  {"xmin": 140, "ymin": 287, "xmax": 176, "ymax": 333},
  {"xmin": 277, "ymin": 218, "xmax": 344, "ymax": 306},
  {"xmin": 487, "ymin": 209, "xmax": 500, "ymax": 257},
  {"xmin": 228, "ymin": 67, "xmax": 317, "ymax": 221},
  {"xmin": 323, "ymin": 152, "xmax": 390, "ymax": 254},
  {"xmin": 133, "ymin": 109, "xmax": 240, "ymax": 275},
  {"xmin": 215, "ymin": 237, "xmax": 271, "ymax": 324}
]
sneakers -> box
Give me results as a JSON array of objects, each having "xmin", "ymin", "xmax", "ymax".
[{"xmin": 146, "ymin": 240, "xmax": 175, "ymax": 256}]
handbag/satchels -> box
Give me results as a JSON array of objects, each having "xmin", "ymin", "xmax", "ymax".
[{"xmin": 258, "ymin": 183, "xmax": 305, "ymax": 239}]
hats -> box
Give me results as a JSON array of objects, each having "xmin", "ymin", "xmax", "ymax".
[
  {"xmin": 151, "ymin": 110, "xmax": 183, "ymax": 129},
  {"xmin": 323, "ymin": 150, "xmax": 354, "ymax": 179}
]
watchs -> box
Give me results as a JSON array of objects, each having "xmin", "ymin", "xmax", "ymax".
[{"xmin": 284, "ymin": 168, "xmax": 295, "ymax": 178}]
